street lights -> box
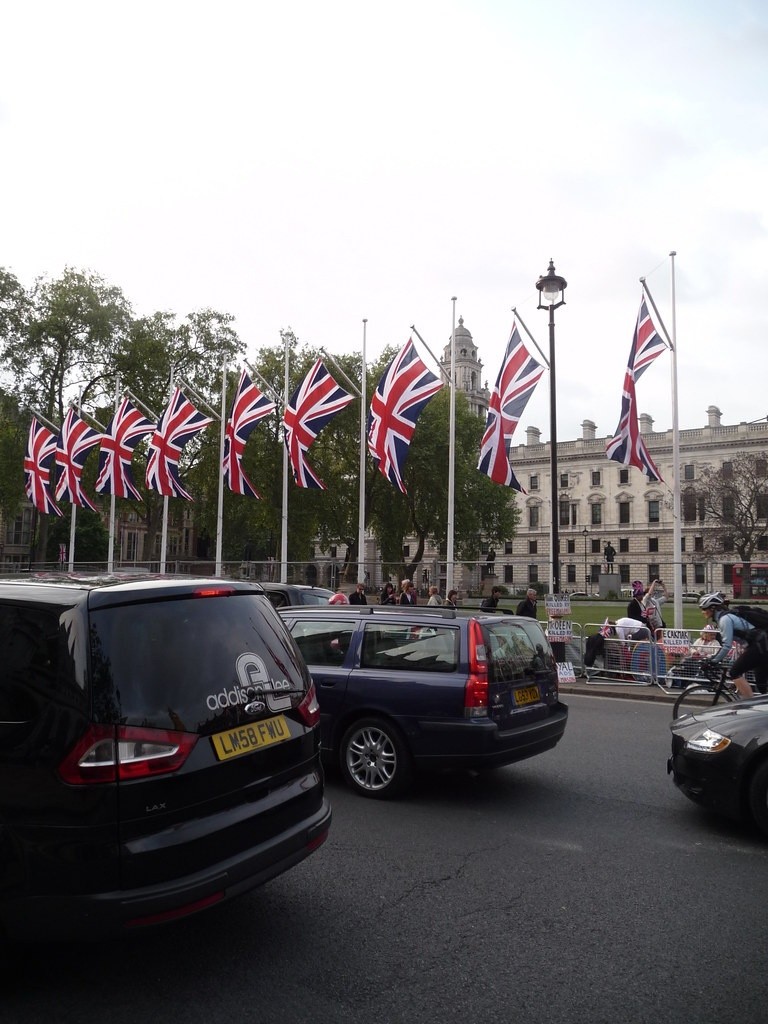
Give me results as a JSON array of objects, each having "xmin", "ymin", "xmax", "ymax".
[
  {"xmin": 265, "ymin": 530, "xmax": 274, "ymax": 582},
  {"xmin": 534, "ymin": 256, "xmax": 568, "ymax": 663},
  {"xmin": 582, "ymin": 526, "xmax": 588, "ymax": 597}
]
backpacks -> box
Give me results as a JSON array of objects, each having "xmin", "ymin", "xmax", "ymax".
[{"xmin": 719, "ymin": 606, "xmax": 768, "ymax": 632}]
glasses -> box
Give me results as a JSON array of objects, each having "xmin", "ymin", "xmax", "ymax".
[{"xmin": 359, "ymin": 588, "xmax": 364, "ymax": 590}]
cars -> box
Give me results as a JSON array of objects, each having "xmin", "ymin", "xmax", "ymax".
[
  {"xmin": 569, "ymin": 592, "xmax": 592, "ymax": 598},
  {"xmin": 681, "ymin": 592, "xmax": 701, "ymax": 603},
  {"xmin": 666, "ymin": 693, "xmax": 768, "ymax": 841},
  {"xmin": 621, "ymin": 589, "xmax": 635, "ymax": 597}
]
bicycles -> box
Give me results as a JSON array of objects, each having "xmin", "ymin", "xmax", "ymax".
[{"xmin": 671, "ymin": 656, "xmax": 758, "ymax": 721}]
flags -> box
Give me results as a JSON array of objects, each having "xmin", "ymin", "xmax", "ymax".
[
  {"xmin": 476, "ymin": 321, "xmax": 545, "ymax": 494},
  {"xmin": 145, "ymin": 387, "xmax": 213, "ymax": 502},
  {"xmin": 605, "ymin": 290, "xmax": 668, "ymax": 481},
  {"xmin": 224, "ymin": 369, "xmax": 277, "ymax": 500},
  {"xmin": 53, "ymin": 407, "xmax": 103, "ymax": 512},
  {"xmin": 23, "ymin": 417, "xmax": 65, "ymax": 517},
  {"xmin": 282, "ymin": 357, "xmax": 354, "ymax": 489},
  {"xmin": 367, "ymin": 338, "xmax": 445, "ymax": 495},
  {"xmin": 95, "ymin": 398, "xmax": 157, "ymax": 500}
]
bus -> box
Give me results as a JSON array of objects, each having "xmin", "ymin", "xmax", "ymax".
[{"xmin": 730, "ymin": 563, "xmax": 768, "ymax": 599}]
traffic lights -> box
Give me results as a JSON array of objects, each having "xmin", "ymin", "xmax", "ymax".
[{"xmin": 586, "ymin": 575, "xmax": 590, "ymax": 583}]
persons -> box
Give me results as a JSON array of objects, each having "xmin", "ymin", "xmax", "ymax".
[
  {"xmin": 610, "ymin": 578, "xmax": 669, "ymax": 647},
  {"xmin": 444, "ymin": 589, "xmax": 458, "ymax": 610},
  {"xmin": 399, "ymin": 579, "xmax": 417, "ymax": 605},
  {"xmin": 516, "ymin": 589, "xmax": 538, "ymax": 619},
  {"xmin": 487, "ymin": 548, "xmax": 496, "ymax": 573},
  {"xmin": 691, "ymin": 592, "xmax": 768, "ymax": 698},
  {"xmin": 427, "ymin": 586, "xmax": 443, "ymax": 605},
  {"xmin": 480, "ymin": 586, "xmax": 501, "ymax": 613},
  {"xmin": 349, "ymin": 584, "xmax": 368, "ymax": 605},
  {"xmin": 604, "ymin": 541, "xmax": 616, "ymax": 574},
  {"xmin": 381, "ymin": 583, "xmax": 396, "ymax": 605}
]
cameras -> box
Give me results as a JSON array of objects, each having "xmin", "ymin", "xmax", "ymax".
[{"xmin": 656, "ymin": 579, "xmax": 663, "ymax": 584}]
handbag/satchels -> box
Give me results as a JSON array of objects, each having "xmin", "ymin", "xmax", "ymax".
[{"xmin": 663, "ymin": 621, "xmax": 667, "ymax": 628}]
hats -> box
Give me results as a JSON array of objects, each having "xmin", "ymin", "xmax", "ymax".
[
  {"xmin": 704, "ymin": 625, "xmax": 716, "ymax": 633},
  {"xmin": 632, "ymin": 581, "xmax": 644, "ymax": 597}
]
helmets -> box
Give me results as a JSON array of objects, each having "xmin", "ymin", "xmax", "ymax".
[{"xmin": 699, "ymin": 592, "xmax": 724, "ymax": 609}]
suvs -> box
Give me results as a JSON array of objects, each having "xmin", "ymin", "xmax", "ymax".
[
  {"xmin": 0, "ymin": 567, "xmax": 333, "ymax": 934},
  {"xmin": 274, "ymin": 603, "xmax": 570, "ymax": 801},
  {"xmin": 258, "ymin": 581, "xmax": 338, "ymax": 607}
]
